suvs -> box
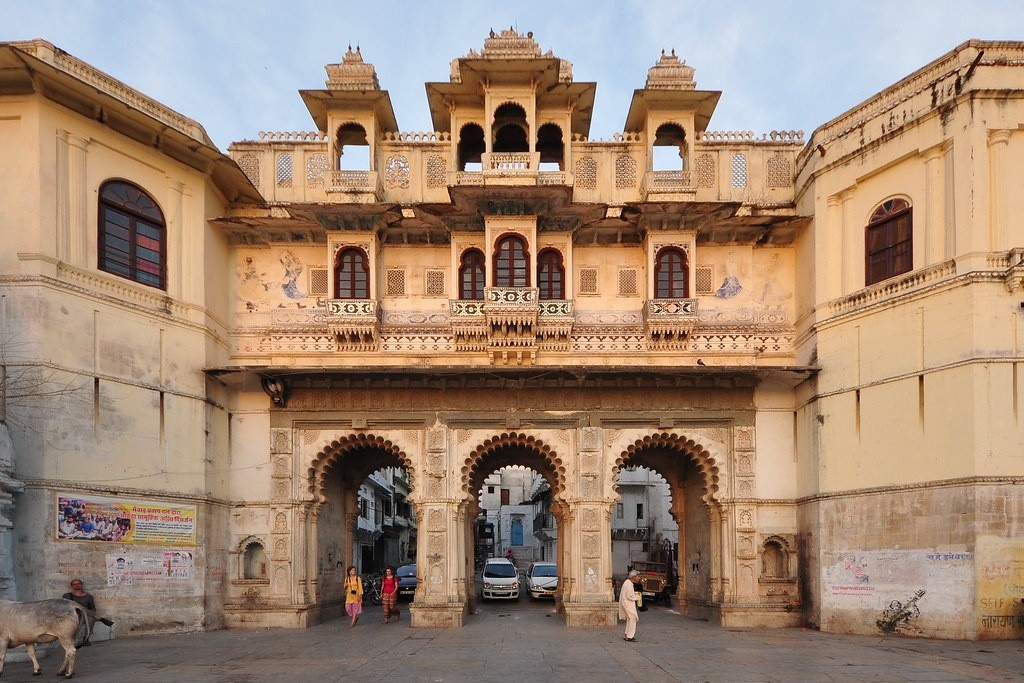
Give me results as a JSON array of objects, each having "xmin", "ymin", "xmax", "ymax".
[{"xmin": 627, "ymin": 559, "xmax": 672, "ymax": 608}]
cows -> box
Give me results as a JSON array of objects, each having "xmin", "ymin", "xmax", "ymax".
[{"xmin": 0, "ymin": 598, "xmax": 115, "ymax": 679}]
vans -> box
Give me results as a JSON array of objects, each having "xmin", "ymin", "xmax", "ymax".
[{"xmin": 481, "ymin": 558, "xmax": 519, "ymax": 602}]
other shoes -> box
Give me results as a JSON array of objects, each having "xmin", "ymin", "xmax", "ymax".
[
  {"xmin": 394, "ymin": 616, "xmax": 400, "ymax": 621},
  {"xmin": 347, "ymin": 624, "xmax": 355, "ymax": 628},
  {"xmin": 83, "ymin": 637, "xmax": 91, "ymax": 646},
  {"xmin": 625, "ymin": 638, "xmax": 638, "ymax": 642},
  {"xmin": 356, "ymin": 617, "xmax": 359, "ymax": 622},
  {"xmin": 623, "ymin": 636, "xmax": 627, "ymax": 640},
  {"xmin": 381, "ymin": 620, "xmax": 388, "ymax": 624}
]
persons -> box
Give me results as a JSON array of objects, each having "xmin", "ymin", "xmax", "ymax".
[
  {"xmin": 505, "ymin": 550, "xmax": 513, "ymax": 560},
  {"xmin": 619, "ymin": 570, "xmax": 641, "ymax": 642},
  {"xmin": 62, "ymin": 579, "xmax": 96, "ymax": 648},
  {"xmin": 59, "ymin": 499, "xmax": 130, "ymax": 541},
  {"xmin": 380, "ymin": 567, "xmax": 400, "ymax": 624},
  {"xmin": 344, "ymin": 566, "xmax": 364, "ymax": 627}
]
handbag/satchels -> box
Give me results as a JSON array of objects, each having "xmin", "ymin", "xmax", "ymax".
[{"xmin": 637, "ymin": 592, "xmax": 642, "ymax": 607}]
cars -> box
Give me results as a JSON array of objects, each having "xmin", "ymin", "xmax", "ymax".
[
  {"xmin": 525, "ymin": 561, "xmax": 558, "ymax": 602},
  {"xmin": 396, "ymin": 564, "xmax": 418, "ymax": 600}
]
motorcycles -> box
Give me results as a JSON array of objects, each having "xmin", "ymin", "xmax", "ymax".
[{"xmin": 361, "ymin": 575, "xmax": 390, "ymax": 608}]
